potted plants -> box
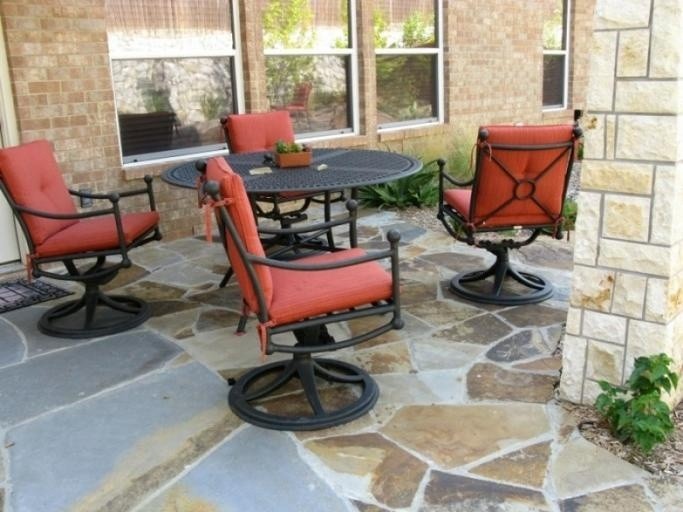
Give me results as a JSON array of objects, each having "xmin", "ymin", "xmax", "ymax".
[{"xmin": 272, "ymin": 139, "xmax": 317, "ymax": 171}]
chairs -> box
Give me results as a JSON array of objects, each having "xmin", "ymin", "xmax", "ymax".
[
  {"xmin": 194, "ymin": 155, "xmax": 405, "ymax": 432},
  {"xmin": 436, "ymin": 120, "xmax": 585, "ymax": 307},
  {"xmin": 1, "ymin": 137, "xmax": 165, "ymax": 341},
  {"xmin": 220, "ymin": 109, "xmax": 325, "ymax": 254},
  {"xmin": 270, "ymin": 81, "xmax": 313, "ymax": 130}
]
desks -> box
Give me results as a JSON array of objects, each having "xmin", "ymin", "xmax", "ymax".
[{"xmin": 161, "ymin": 147, "xmax": 426, "ymax": 338}]
